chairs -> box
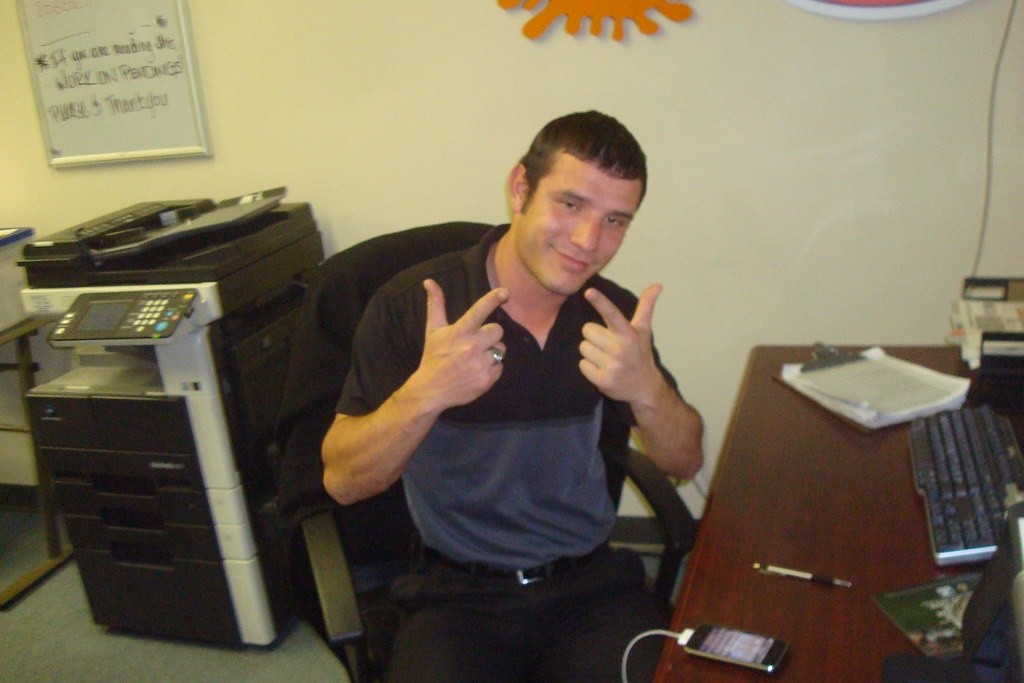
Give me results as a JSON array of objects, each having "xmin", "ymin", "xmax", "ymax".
[{"xmin": 289, "ymin": 221, "xmax": 699, "ymax": 683}]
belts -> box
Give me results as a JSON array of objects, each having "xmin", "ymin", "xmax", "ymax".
[{"xmin": 426, "ymin": 538, "xmax": 610, "ymax": 579}]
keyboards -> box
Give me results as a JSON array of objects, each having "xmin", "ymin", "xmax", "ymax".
[{"xmin": 910, "ymin": 403, "xmax": 1024, "ymax": 564}]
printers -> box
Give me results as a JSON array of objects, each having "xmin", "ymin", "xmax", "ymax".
[{"xmin": 16, "ymin": 186, "xmax": 326, "ymax": 652}]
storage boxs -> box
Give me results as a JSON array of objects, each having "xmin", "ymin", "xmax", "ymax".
[
  {"xmin": 25, "ymin": 392, "xmax": 279, "ymax": 646},
  {"xmin": 0, "ymin": 227, "xmax": 37, "ymax": 334}
]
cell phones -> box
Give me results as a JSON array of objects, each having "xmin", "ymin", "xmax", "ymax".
[{"xmin": 684, "ymin": 624, "xmax": 789, "ymax": 673}]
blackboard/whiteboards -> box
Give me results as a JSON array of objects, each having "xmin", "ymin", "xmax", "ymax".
[{"xmin": 15, "ymin": 1, "xmax": 212, "ymax": 169}]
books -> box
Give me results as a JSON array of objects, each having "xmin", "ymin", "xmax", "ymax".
[{"xmin": 951, "ymin": 299, "xmax": 1024, "ymax": 369}]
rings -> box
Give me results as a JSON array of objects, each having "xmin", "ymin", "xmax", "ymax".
[{"xmin": 489, "ymin": 347, "xmax": 505, "ymax": 362}]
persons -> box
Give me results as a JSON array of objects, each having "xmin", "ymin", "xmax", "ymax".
[{"xmin": 321, "ymin": 110, "xmax": 705, "ymax": 683}]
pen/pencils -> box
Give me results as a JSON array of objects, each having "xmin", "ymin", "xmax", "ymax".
[{"xmin": 752, "ymin": 563, "xmax": 852, "ymax": 590}]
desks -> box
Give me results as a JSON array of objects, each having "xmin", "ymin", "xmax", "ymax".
[
  {"xmin": 0, "ymin": 315, "xmax": 77, "ymax": 607},
  {"xmin": 652, "ymin": 344, "xmax": 1024, "ymax": 683}
]
